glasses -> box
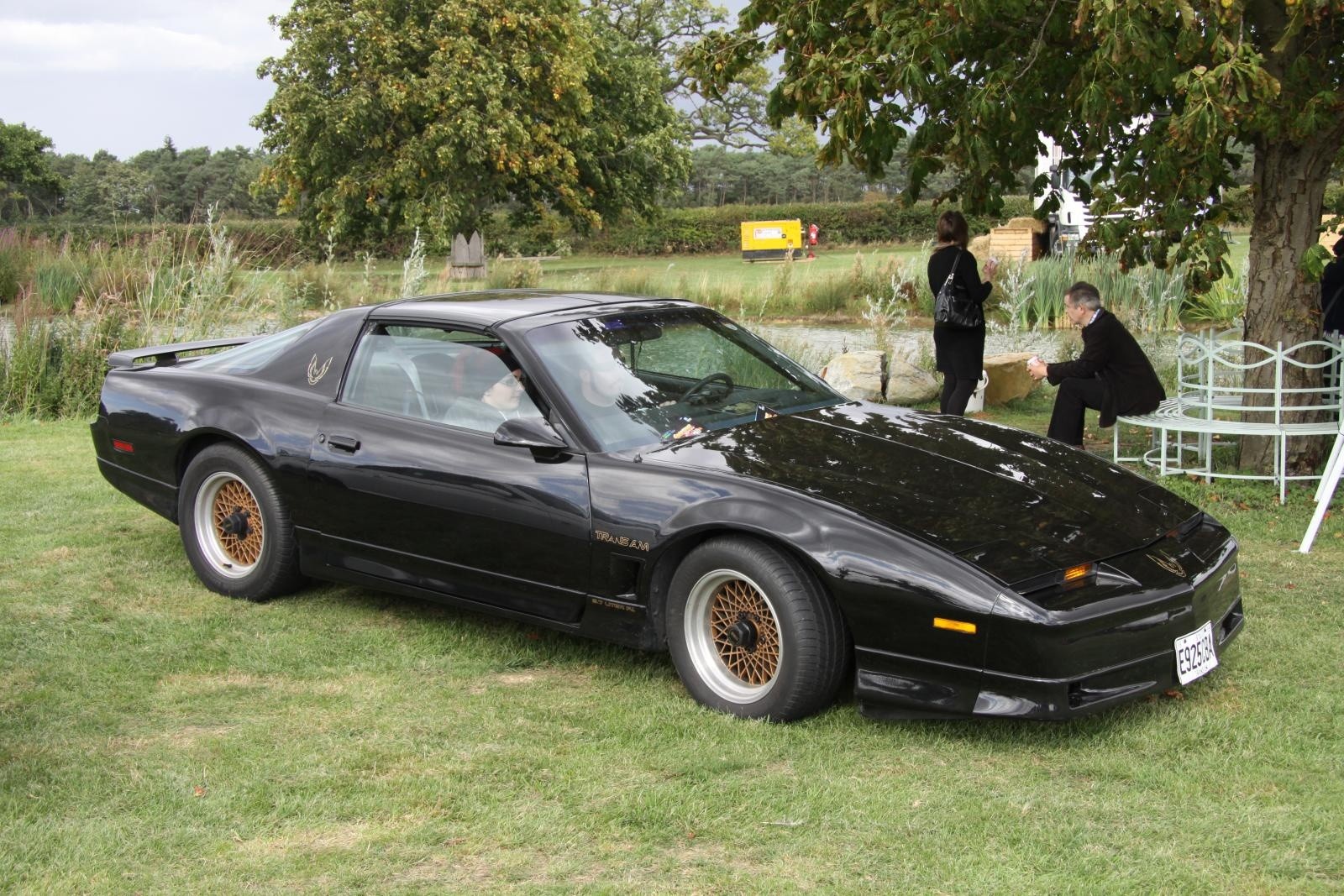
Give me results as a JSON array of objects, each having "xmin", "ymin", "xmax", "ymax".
[{"xmin": 499, "ymin": 377, "xmax": 526, "ymax": 387}]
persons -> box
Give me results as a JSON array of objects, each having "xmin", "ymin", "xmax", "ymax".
[
  {"xmin": 1025, "ymin": 280, "xmax": 1167, "ymax": 450},
  {"xmin": 433, "ymin": 345, "xmax": 573, "ymax": 452},
  {"xmin": 556, "ymin": 327, "xmax": 676, "ymax": 434},
  {"xmin": 1319, "ymin": 236, "xmax": 1344, "ymax": 402},
  {"xmin": 927, "ymin": 209, "xmax": 998, "ymax": 417}
]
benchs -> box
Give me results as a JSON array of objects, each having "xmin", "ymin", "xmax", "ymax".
[
  {"xmin": 1114, "ymin": 316, "xmax": 1344, "ymax": 505},
  {"xmin": 350, "ymin": 351, "xmax": 457, "ymax": 423}
]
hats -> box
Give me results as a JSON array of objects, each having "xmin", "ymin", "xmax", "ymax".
[{"xmin": 470, "ymin": 347, "xmax": 520, "ymax": 394}]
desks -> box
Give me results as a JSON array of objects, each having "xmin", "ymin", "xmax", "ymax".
[{"xmin": 1220, "ymin": 230, "xmax": 1232, "ymax": 242}]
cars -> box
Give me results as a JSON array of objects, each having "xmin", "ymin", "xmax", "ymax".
[{"xmin": 89, "ymin": 285, "xmax": 1247, "ymax": 723}]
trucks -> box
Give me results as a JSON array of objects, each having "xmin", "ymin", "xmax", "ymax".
[{"xmin": 1030, "ymin": 111, "xmax": 1154, "ymax": 262}]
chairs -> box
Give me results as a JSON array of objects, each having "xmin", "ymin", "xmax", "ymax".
[{"xmin": 438, "ymin": 345, "xmax": 505, "ymax": 420}]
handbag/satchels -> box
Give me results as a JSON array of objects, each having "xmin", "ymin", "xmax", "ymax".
[{"xmin": 936, "ymin": 249, "xmax": 982, "ymax": 329}]
